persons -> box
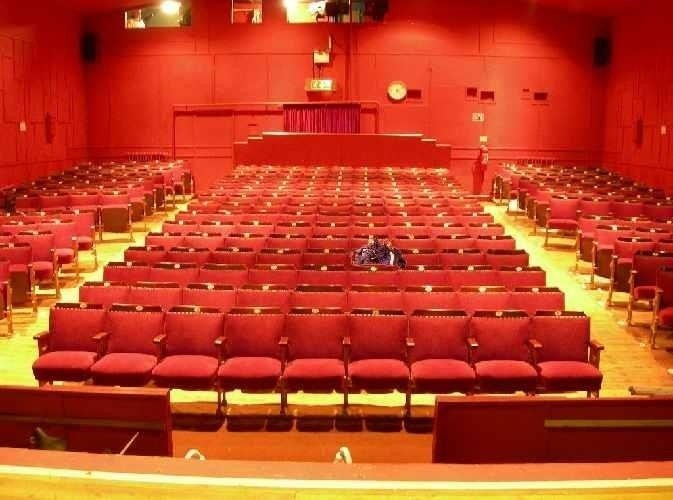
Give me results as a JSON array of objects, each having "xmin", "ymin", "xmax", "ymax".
[{"xmin": 127, "ymin": 9, "xmax": 145, "ymax": 29}]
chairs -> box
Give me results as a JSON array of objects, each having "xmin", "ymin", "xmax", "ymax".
[{"xmin": 0, "ymin": 160, "xmax": 673, "ymax": 418}]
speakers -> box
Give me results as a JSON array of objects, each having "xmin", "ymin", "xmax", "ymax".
[
  {"xmin": 592, "ymin": 37, "xmax": 611, "ymax": 64},
  {"xmin": 80, "ymin": 31, "xmax": 97, "ymax": 64}
]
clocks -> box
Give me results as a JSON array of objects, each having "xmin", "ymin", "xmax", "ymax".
[{"xmin": 386, "ymin": 81, "xmax": 407, "ymax": 101}]
